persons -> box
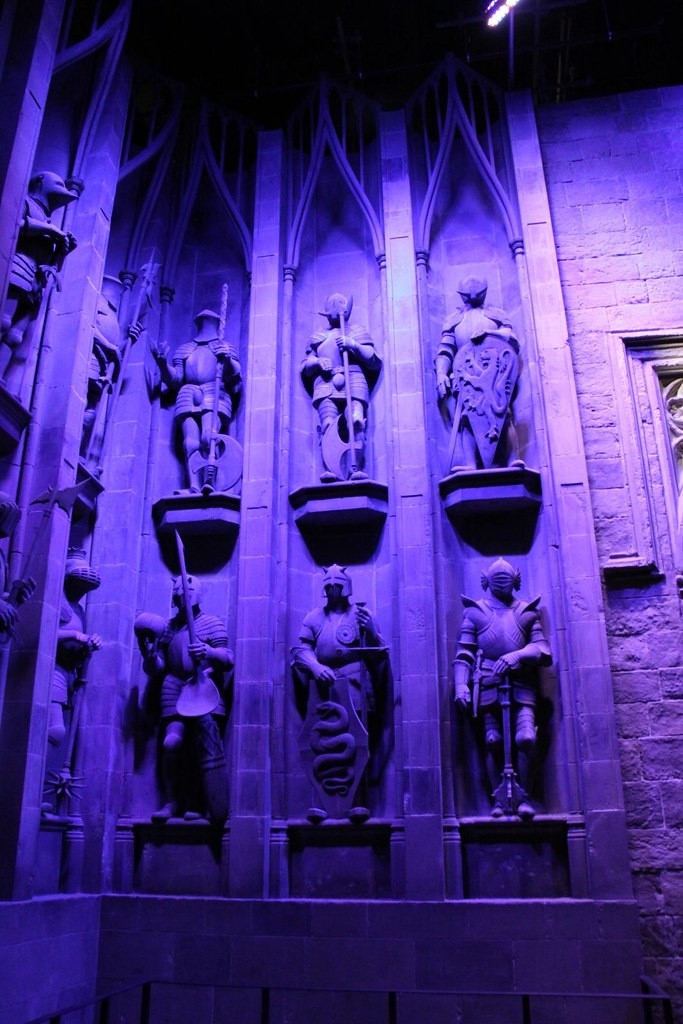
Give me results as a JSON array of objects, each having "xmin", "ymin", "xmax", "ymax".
[
  {"xmin": 132, "ymin": 556, "xmax": 557, "ymax": 826},
  {"xmin": 1, "ymin": 170, "xmax": 145, "ymax": 462},
  {"xmin": 152, "ymin": 274, "xmax": 526, "ymax": 495},
  {"xmin": 2, "ymin": 491, "xmax": 109, "ymax": 818}
]
